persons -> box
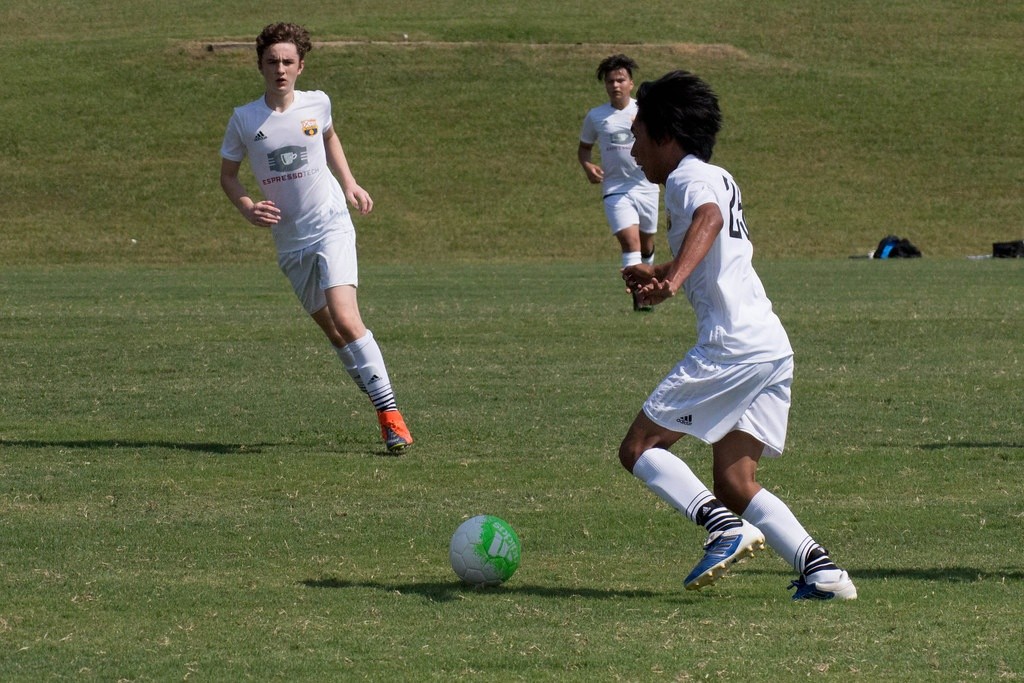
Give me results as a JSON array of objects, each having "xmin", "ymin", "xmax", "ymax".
[
  {"xmin": 619, "ymin": 69, "xmax": 857, "ymax": 599},
  {"xmin": 219, "ymin": 21, "xmax": 413, "ymax": 456},
  {"xmin": 578, "ymin": 55, "xmax": 660, "ymax": 311}
]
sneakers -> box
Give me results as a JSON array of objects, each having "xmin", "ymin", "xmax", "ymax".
[
  {"xmin": 378, "ymin": 410, "xmax": 413, "ymax": 456},
  {"xmin": 785, "ymin": 570, "xmax": 857, "ymax": 601},
  {"xmin": 684, "ymin": 518, "xmax": 766, "ymax": 592}
]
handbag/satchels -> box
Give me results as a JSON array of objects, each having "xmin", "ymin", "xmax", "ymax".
[
  {"xmin": 992, "ymin": 240, "xmax": 1024, "ymax": 258},
  {"xmin": 873, "ymin": 235, "xmax": 921, "ymax": 259}
]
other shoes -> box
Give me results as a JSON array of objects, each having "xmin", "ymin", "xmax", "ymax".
[{"xmin": 633, "ymin": 288, "xmax": 654, "ymax": 312}]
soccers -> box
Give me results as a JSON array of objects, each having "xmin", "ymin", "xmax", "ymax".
[{"xmin": 446, "ymin": 511, "xmax": 524, "ymax": 591}]
